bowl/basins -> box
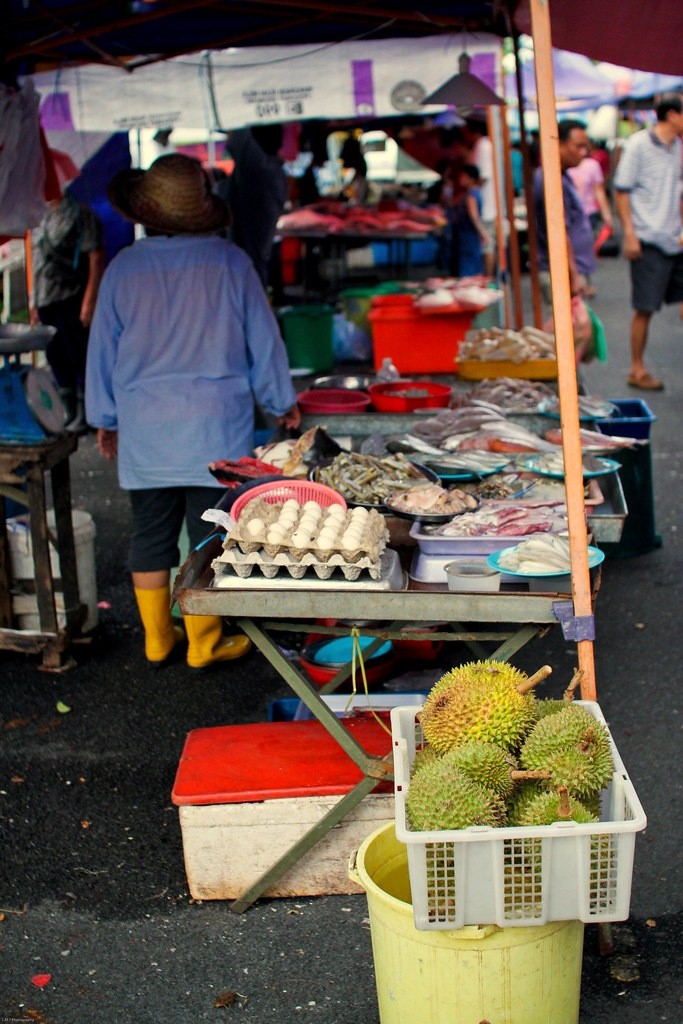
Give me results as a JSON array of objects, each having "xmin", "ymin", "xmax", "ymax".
[
  {"xmin": 368, "ymin": 382, "xmax": 454, "ymax": 412},
  {"xmin": 296, "ymin": 374, "xmax": 387, "ymax": 414},
  {"xmin": 300, "ymin": 636, "xmax": 392, "ymax": 691}
]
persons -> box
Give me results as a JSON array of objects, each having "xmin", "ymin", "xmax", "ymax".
[
  {"xmin": 526, "ymin": 118, "xmax": 612, "ymax": 395},
  {"xmin": 28, "ymin": 197, "xmax": 105, "ymax": 438},
  {"xmin": 86, "ymin": 153, "xmax": 300, "ymax": 667},
  {"xmin": 509, "ymin": 146, "xmax": 522, "ymax": 198},
  {"xmin": 614, "ymin": 91, "xmax": 683, "ymax": 390},
  {"xmin": 431, "ymin": 114, "xmax": 498, "ymax": 279},
  {"xmin": 205, "ymin": 121, "xmax": 286, "ymax": 326}
]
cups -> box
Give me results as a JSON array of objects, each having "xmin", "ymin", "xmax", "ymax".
[{"xmin": 445, "ymin": 559, "xmax": 501, "ymax": 593}]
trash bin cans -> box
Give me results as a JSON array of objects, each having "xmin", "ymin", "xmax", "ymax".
[{"xmin": 596, "ymin": 397, "xmax": 663, "ymax": 557}]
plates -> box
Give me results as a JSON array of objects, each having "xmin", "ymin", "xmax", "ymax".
[
  {"xmin": 537, "ymin": 397, "xmax": 613, "ymax": 419},
  {"xmin": 524, "ymin": 456, "xmax": 621, "ymax": 476},
  {"xmin": 307, "ymin": 448, "xmax": 513, "ymax": 522},
  {"xmin": 485, "ymin": 545, "xmax": 604, "ymax": 577}
]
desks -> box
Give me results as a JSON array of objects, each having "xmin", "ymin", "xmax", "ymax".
[{"xmin": 0, "ymin": 431, "xmax": 89, "ymax": 672}]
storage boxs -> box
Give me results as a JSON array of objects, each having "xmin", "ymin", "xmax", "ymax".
[
  {"xmin": 170, "ymin": 693, "xmax": 427, "ymax": 899},
  {"xmin": 391, "ymin": 696, "xmax": 647, "ymax": 931},
  {"xmin": 279, "ymin": 275, "xmax": 501, "ymax": 378}
]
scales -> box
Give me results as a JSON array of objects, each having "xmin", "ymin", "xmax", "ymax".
[{"xmin": 0, "ymin": 323, "xmax": 68, "ymax": 445}]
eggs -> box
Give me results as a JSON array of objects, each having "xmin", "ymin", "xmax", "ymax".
[{"xmin": 247, "ymin": 499, "xmax": 368, "ymax": 549}]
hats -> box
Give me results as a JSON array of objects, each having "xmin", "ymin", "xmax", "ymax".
[{"xmin": 105, "ymin": 153, "xmax": 232, "ymax": 235}]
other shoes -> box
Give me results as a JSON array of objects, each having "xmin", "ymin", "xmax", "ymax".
[{"xmin": 628, "ymin": 370, "xmax": 664, "ymax": 389}]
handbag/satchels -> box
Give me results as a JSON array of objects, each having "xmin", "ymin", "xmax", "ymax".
[
  {"xmin": 594, "ymin": 224, "xmax": 621, "ymax": 259},
  {"xmin": 540, "ymin": 293, "xmax": 607, "ymax": 367}
]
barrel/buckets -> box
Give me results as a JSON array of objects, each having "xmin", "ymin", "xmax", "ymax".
[
  {"xmin": 347, "ymin": 820, "xmax": 585, "ymax": 1024},
  {"xmin": 5, "ymin": 507, "xmax": 98, "ymax": 633}
]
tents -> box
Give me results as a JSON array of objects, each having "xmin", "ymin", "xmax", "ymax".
[
  {"xmin": 14, "ymin": 36, "xmax": 683, "ymax": 139},
  {"xmin": 0, "ymin": 0, "xmax": 683, "ymax": 723}
]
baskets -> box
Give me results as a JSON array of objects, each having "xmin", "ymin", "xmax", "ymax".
[
  {"xmin": 231, "ymin": 479, "xmax": 348, "ymax": 530},
  {"xmin": 391, "ymin": 699, "xmax": 647, "ymax": 931}
]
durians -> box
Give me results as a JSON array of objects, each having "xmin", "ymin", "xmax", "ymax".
[{"xmin": 405, "ymin": 658, "xmax": 616, "ymax": 910}]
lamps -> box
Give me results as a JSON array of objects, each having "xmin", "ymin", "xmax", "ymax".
[{"xmin": 421, "ymin": 18, "xmax": 509, "ymax": 116}]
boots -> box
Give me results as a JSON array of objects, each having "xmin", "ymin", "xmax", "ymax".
[
  {"xmin": 174, "ymin": 587, "xmax": 249, "ymax": 669},
  {"xmin": 62, "ymin": 390, "xmax": 93, "ymax": 435},
  {"xmin": 132, "ymin": 587, "xmax": 183, "ymax": 667}
]
